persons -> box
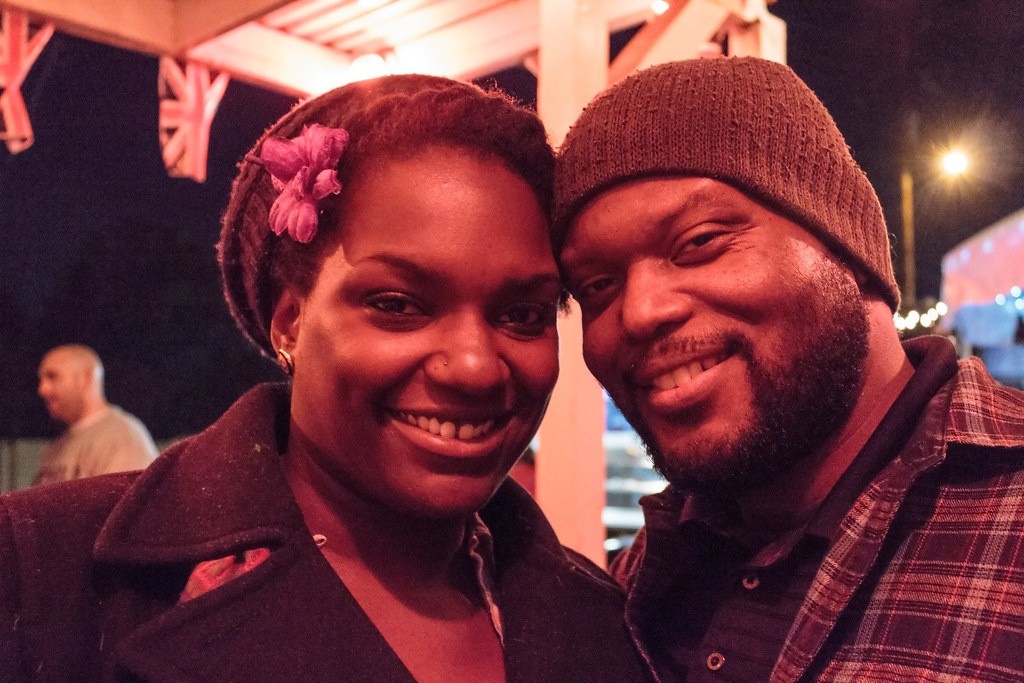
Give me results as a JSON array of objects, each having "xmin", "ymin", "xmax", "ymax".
[
  {"xmin": 555, "ymin": 55, "xmax": 1024, "ymax": 683},
  {"xmin": 30, "ymin": 343, "xmax": 161, "ymax": 489},
  {"xmin": 0, "ymin": 75, "xmax": 660, "ymax": 683}
]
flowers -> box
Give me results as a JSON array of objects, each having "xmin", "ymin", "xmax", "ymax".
[{"xmin": 257, "ymin": 121, "xmax": 351, "ymax": 247}]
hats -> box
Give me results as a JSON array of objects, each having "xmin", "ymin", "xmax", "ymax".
[{"xmin": 548, "ymin": 57, "xmax": 901, "ymax": 315}]
